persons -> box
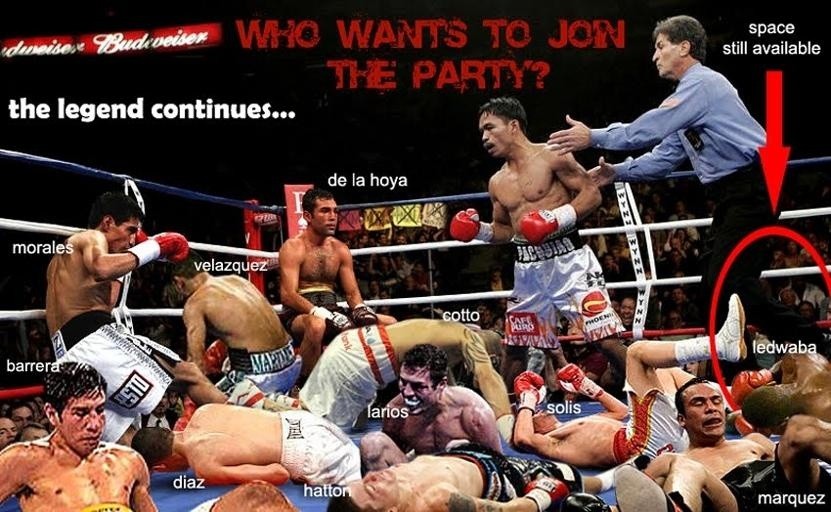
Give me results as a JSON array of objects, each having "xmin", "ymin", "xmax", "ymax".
[
  {"xmin": 278, "ymin": 187, "xmax": 400, "ymax": 390},
  {"xmin": 45, "ymin": 190, "xmax": 232, "ymax": 449},
  {"xmin": 1, "ymin": 316, "xmax": 831, "ymax": 509},
  {"xmin": 450, "ymin": 95, "xmax": 629, "ymax": 400},
  {"xmin": 159, "ymin": 247, "xmax": 307, "ymax": 398},
  {"xmin": 338, "ymin": 169, "xmax": 831, "ymax": 315},
  {"xmin": 546, "ymin": 14, "xmax": 831, "ymax": 380}
]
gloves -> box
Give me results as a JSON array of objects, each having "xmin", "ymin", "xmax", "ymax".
[
  {"xmin": 514, "ymin": 371, "xmax": 546, "ymax": 411},
  {"xmin": 152, "ymin": 231, "xmax": 189, "ymax": 261},
  {"xmin": 558, "ymin": 362, "xmax": 602, "ymax": 401},
  {"xmin": 560, "ymin": 492, "xmax": 609, "ymax": 512},
  {"xmin": 326, "ymin": 311, "xmax": 353, "ymax": 331},
  {"xmin": 526, "ymin": 476, "xmax": 566, "ymax": 503},
  {"xmin": 521, "ymin": 210, "xmax": 557, "ymax": 244},
  {"xmin": 205, "ymin": 339, "xmax": 227, "ymax": 374},
  {"xmin": 351, "ymin": 304, "xmax": 377, "ymax": 325},
  {"xmin": 730, "ymin": 369, "xmax": 775, "ymax": 406},
  {"xmin": 450, "ymin": 208, "xmax": 480, "ymax": 242}
]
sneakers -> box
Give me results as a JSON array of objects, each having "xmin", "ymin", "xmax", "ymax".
[
  {"xmin": 718, "ymin": 294, "xmax": 749, "ymax": 365},
  {"xmin": 614, "ymin": 465, "xmax": 680, "ymax": 512},
  {"xmin": 226, "ymin": 380, "xmax": 267, "ymax": 408}
]
